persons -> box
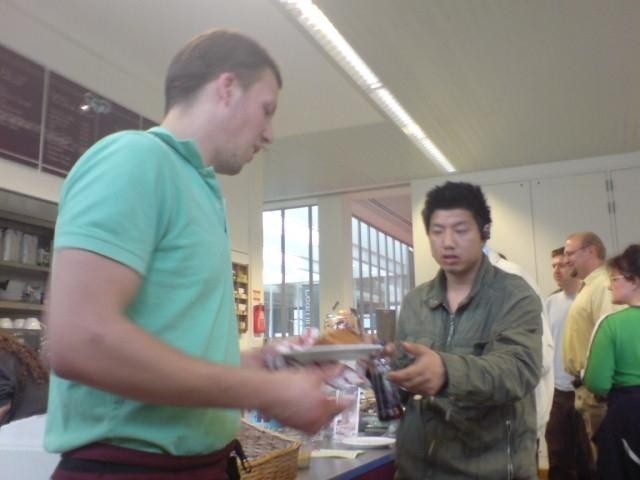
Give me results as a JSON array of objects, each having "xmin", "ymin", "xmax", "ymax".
[
  {"xmin": 562, "ymin": 233, "xmax": 628, "ymax": 480},
  {"xmin": 539, "ymin": 246, "xmax": 585, "ymax": 479},
  {"xmin": 382, "ymin": 180, "xmax": 544, "ymax": 479},
  {"xmin": 481, "ymin": 203, "xmax": 555, "ymax": 479},
  {"xmin": 41, "ymin": 29, "xmax": 355, "ymax": 479},
  {"xmin": 579, "ymin": 244, "xmax": 640, "ymax": 480}
]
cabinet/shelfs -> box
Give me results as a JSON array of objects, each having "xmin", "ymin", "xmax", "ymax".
[
  {"xmin": 411, "ymin": 150, "xmax": 617, "ymax": 296},
  {"xmin": 0, "ymin": 213, "xmax": 55, "ymax": 337},
  {"xmin": 609, "ymin": 151, "xmax": 640, "ymax": 254},
  {"xmin": 232, "ymin": 251, "xmax": 252, "ymax": 339}
]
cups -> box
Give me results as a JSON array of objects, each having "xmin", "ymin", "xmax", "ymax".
[
  {"xmin": 1, "ymin": 317, "xmax": 46, "ymax": 328},
  {"xmin": 367, "ymin": 349, "xmax": 406, "ymax": 423},
  {"xmin": 285, "ymin": 430, "xmax": 311, "ymax": 471},
  {"xmin": 335, "ymin": 382, "xmax": 360, "ymax": 439}
]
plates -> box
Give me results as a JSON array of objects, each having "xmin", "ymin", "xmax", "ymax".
[{"xmin": 342, "ymin": 435, "xmax": 396, "ymax": 451}]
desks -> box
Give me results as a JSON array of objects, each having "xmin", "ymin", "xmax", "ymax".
[{"xmin": 295, "ymin": 430, "xmax": 396, "ymax": 480}]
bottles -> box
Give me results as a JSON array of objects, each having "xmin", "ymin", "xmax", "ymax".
[
  {"xmin": 254, "ymin": 304, "xmax": 267, "ymax": 333},
  {"xmin": 324, "ymin": 313, "xmax": 355, "ymax": 334}
]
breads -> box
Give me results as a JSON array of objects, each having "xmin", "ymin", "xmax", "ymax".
[{"xmin": 315, "ymin": 327, "xmax": 364, "ymax": 345}]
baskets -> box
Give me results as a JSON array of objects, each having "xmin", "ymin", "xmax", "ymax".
[{"xmin": 237, "ymin": 418, "xmax": 301, "ymax": 480}]
